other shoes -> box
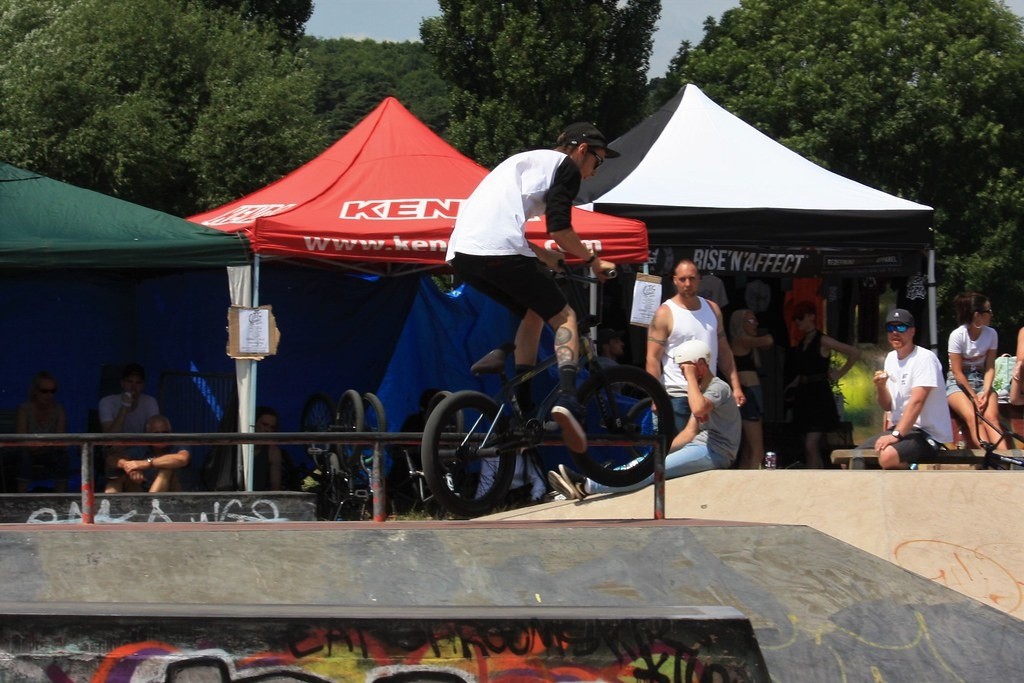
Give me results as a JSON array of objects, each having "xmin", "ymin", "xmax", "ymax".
[{"xmin": 551, "ymin": 394, "xmax": 586, "ymax": 452}]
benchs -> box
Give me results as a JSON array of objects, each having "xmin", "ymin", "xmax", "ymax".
[{"xmin": 831, "ymin": 445, "xmax": 1023, "ymax": 470}]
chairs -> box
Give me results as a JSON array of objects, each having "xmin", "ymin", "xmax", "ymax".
[{"xmin": 0, "ymin": 409, "xmax": 48, "ymax": 493}]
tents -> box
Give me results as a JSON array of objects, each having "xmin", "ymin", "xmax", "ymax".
[
  {"xmin": 187, "ymin": 94, "xmax": 650, "ymax": 491},
  {"xmin": 575, "ymin": 83, "xmax": 938, "ymax": 358},
  {"xmin": 0, "ymin": 158, "xmax": 249, "ymax": 487}
]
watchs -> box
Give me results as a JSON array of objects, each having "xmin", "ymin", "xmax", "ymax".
[
  {"xmin": 892, "ymin": 431, "xmax": 903, "ymax": 440},
  {"xmin": 147, "ymin": 458, "xmax": 153, "ymax": 468}
]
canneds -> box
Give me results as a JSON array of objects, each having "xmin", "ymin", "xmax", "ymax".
[{"xmin": 765, "ymin": 451, "xmax": 776, "ymax": 470}]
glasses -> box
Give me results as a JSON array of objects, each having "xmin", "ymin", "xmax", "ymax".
[
  {"xmin": 981, "ymin": 308, "xmax": 993, "ymax": 316},
  {"xmin": 744, "ymin": 318, "xmax": 756, "ymax": 324},
  {"xmin": 587, "ymin": 146, "xmax": 603, "ymax": 170},
  {"xmin": 37, "ymin": 388, "xmax": 57, "ymax": 395},
  {"xmin": 791, "ymin": 313, "xmax": 805, "ymax": 322},
  {"xmin": 885, "ymin": 323, "xmax": 911, "ymax": 333}
]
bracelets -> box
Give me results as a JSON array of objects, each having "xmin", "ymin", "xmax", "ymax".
[
  {"xmin": 121, "ymin": 400, "xmax": 132, "ymax": 407},
  {"xmin": 585, "ymin": 255, "xmax": 596, "ymax": 264},
  {"xmin": 1013, "ymin": 375, "xmax": 1022, "ymax": 382}
]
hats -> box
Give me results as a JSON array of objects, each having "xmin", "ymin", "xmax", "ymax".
[
  {"xmin": 556, "ymin": 122, "xmax": 621, "ymax": 159},
  {"xmin": 598, "ymin": 328, "xmax": 624, "ymax": 344},
  {"xmin": 120, "ymin": 363, "xmax": 146, "ymax": 381},
  {"xmin": 885, "ymin": 309, "xmax": 914, "ymax": 327}
]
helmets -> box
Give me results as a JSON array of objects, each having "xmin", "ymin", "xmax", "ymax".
[{"xmin": 669, "ymin": 339, "xmax": 711, "ymax": 365}]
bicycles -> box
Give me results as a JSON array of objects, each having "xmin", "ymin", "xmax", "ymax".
[
  {"xmin": 421, "ymin": 270, "xmax": 676, "ymax": 516},
  {"xmin": 302, "ymin": 390, "xmax": 397, "ymax": 521},
  {"xmin": 956, "ymin": 383, "xmax": 1024, "ymax": 472}
]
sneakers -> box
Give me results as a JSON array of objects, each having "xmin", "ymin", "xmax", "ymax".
[
  {"xmin": 547, "ymin": 469, "xmax": 583, "ymax": 500},
  {"xmin": 558, "ymin": 463, "xmax": 590, "ymax": 501}
]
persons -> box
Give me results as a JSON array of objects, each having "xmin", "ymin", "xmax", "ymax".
[
  {"xmin": 1010, "ymin": 327, "xmax": 1024, "ymax": 406},
  {"xmin": 6, "ymin": 372, "xmax": 73, "ymax": 493},
  {"xmin": 548, "ymin": 341, "xmax": 741, "ymax": 500},
  {"xmin": 598, "ymin": 328, "xmax": 627, "ymax": 393},
  {"xmin": 218, "ymin": 407, "xmax": 296, "ymax": 491},
  {"xmin": 851, "ymin": 309, "xmax": 952, "ymax": 469},
  {"xmin": 947, "ymin": 290, "xmax": 1009, "ymax": 469},
  {"xmin": 647, "ymin": 259, "xmax": 745, "ymax": 442},
  {"xmin": 104, "ymin": 416, "xmax": 198, "ymax": 492},
  {"xmin": 698, "ymin": 276, "xmax": 859, "ymax": 469},
  {"xmin": 444, "ymin": 121, "xmax": 620, "ymax": 452},
  {"xmin": 97, "ymin": 362, "xmax": 160, "ymax": 448}
]
